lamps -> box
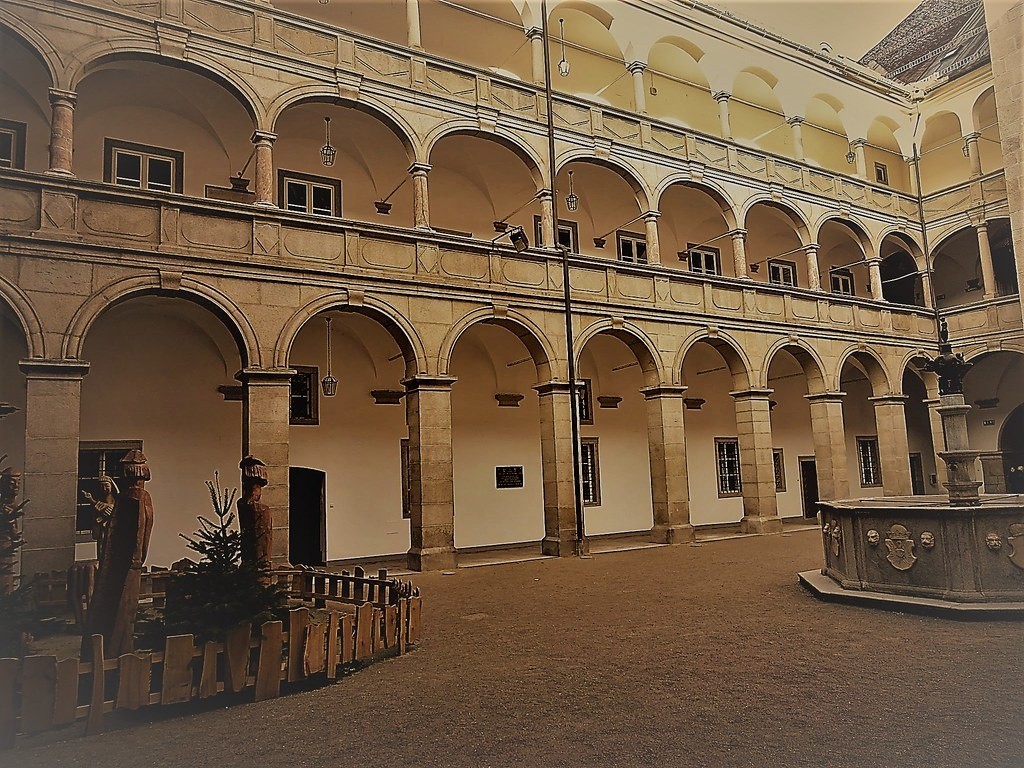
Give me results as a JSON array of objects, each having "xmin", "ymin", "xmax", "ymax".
[
  {"xmin": 845, "ymin": 140, "xmax": 855, "ymax": 163},
  {"xmin": 559, "ymin": 17, "xmax": 570, "ymax": 76},
  {"xmin": 320, "ymin": 318, "xmax": 338, "ymax": 397},
  {"xmin": 963, "ymin": 142, "xmax": 969, "ymax": 157},
  {"xmin": 319, "ymin": 117, "xmax": 336, "ymax": 166},
  {"xmin": 567, "ymin": 169, "xmax": 579, "ymax": 211},
  {"xmin": 491, "ymin": 227, "xmax": 528, "ymax": 254}
]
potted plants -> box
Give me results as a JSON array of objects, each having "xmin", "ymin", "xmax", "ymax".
[{"xmin": 179, "ymin": 474, "xmax": 297, "ymax": 686}]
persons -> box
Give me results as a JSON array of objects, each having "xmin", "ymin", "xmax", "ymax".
[
  {"xmin": 0, "ymin": 466, "xmax": 22, "ymax": 594},
  {"xmin": 82, "ymin": 475, "xmax": 120, "ymax": 561}
]
